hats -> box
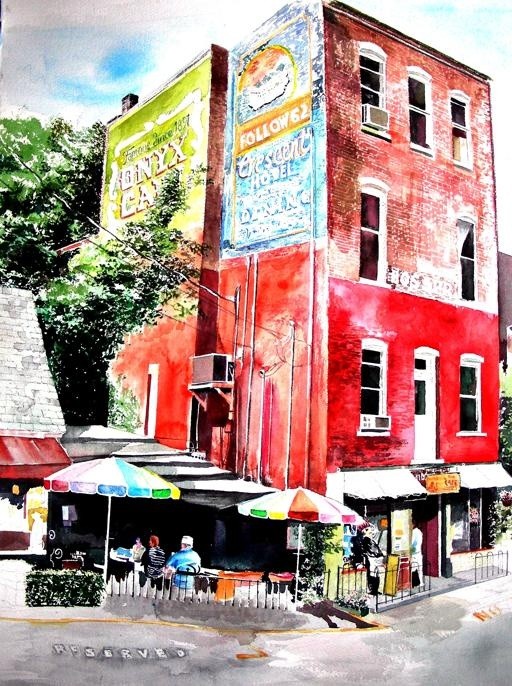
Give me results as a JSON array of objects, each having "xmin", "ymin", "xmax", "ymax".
[{"xmin": 181, "ymin": 535, "xmax": 194, "ymax": 546}]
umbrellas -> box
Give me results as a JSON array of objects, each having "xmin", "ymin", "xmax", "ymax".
[
  {"xmin": 45, "ymin": 453, "xmax": 181, "ymax": 575},
  {"xmin": 237, "ymin": 484, "xmax": 366, "ymax": 608}
]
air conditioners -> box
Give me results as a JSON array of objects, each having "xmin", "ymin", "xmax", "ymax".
[
  {"xmin": 192, "ymin": 353, "xmax": 233, "ymax": 382},
  {"xmin": 360, "ymin": 415, "xmax": 391, "ymax": 430},
  {"xmin": 362, "ymin": 103, "xmax": 389, "ymax": 131}
]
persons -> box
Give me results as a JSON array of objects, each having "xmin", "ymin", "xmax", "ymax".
[
  {"xmin": 163, "ymin": 535, "xmax": 202, "ymax": 590},
  {"xmin": 408, "ymin": 518, "xmax": 425, "ymax": 588},
  {"xmin": 138, "ymin": 536, "xmax": 166, "ymax": 586},
  {"xmin": 129, "ymin": 534, "xmax": 147, "ymax": 561}
]
